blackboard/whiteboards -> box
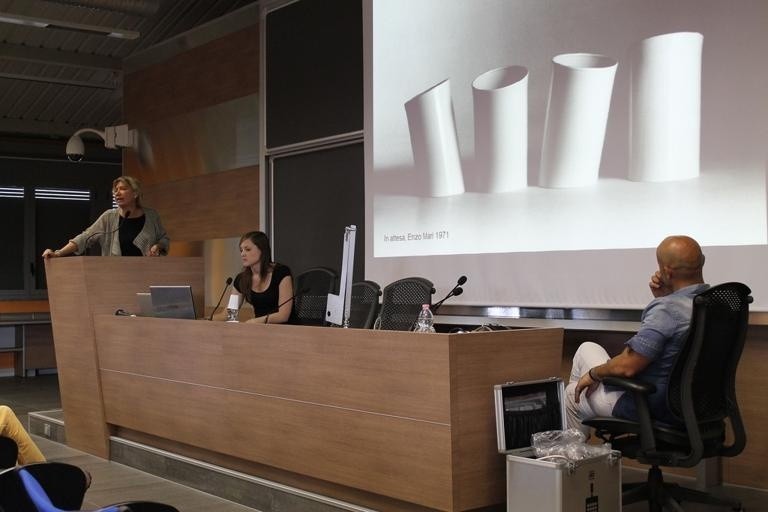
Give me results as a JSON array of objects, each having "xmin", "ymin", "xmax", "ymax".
[{"xmin": 263, "ymin": 0, "xmax": 365, "ymax": 284}]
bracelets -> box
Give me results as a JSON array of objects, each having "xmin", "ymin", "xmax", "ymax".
[
  {"xmin": 592, "ymin": 366, "xmax": 602, "ymax": 381},
  {"xmin": 589, "ymin": 368, "xmax": 595, "ymax": 381},
  {"xmin": 55, "ymin": 250, "xmax": 64, "ymax": 256}
]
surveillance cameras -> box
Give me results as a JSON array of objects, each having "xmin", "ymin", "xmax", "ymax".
[{"xmin": 66, "ymin": 136, "xmax": 85, "ymax": 165}]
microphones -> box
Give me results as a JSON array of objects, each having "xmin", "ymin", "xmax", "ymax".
[
  {"xmin": 82, "ymin": 209, "xmax": 131, "ymax": 255},
  {"xmin": 209, "ymin": 277, "xmax": 233, "ymax": 321},
  {"xmin": 409, "ymin": 287, "xmax": 462, "ymax": 333},
  {"xmin": 431, "ymin": 275, "xmax": 467, "ymax": 316},
  {"xmin": 263, "ymin": 286, "xmax": 310, "ymax": 323}
]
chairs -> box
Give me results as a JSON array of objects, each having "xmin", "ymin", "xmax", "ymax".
[
  {"xmin": 374, "ymin": 277, "xmax": 437, "ymax": 334},
  {"xmin": 0, "ymin": 459, "xmax": 92, "ymax": 512},
  {"xmin": 1, "ymin": 435, "xmax": 18, "ymax": 471},
  {"xmin": 581, "ymin": 281, "xmax": 752, "ymax": 512},
  {"xmin": 95, "ymin": 500, "xmax": 179, "ymax": 512},
  {"xmin": 328, "ymin": 283, "xmax": 382, "ymax": 330},
  {"xmin": 282, "ymin": 266, "xmax": 339, "ymax": 327}
]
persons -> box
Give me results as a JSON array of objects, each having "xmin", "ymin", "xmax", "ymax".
[
  {"xmin": 561, "ymin": 235, "xmax": 711, "ymax": 444},
  {"xmin": 202, "ymin": 231, "xmax": 295, "ymax": 324},
  {"xmin": 42, "ymin": 176, "xmax": 170, "ymax": 257},
  {"xmin": 0, "ymin": 404, "xmax": 92, "ymax": 492}
]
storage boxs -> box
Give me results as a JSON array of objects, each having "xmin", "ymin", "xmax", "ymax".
[{"xmin": 492, "ymin": 377, "xmax": 622, "ymax": 511}]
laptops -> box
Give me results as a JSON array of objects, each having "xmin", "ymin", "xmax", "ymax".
[
  {"xmin": 134, "ymin": 293, "xmax": 151, "ymax": 319},
  {"xmin": 149, "ymin": 285, "xmax": 197, "ymax": 321}
]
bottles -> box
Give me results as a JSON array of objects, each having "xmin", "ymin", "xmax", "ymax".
[{"xmin": 418, "ymin": 304, "xmax": 436, "ymax": 333}]
